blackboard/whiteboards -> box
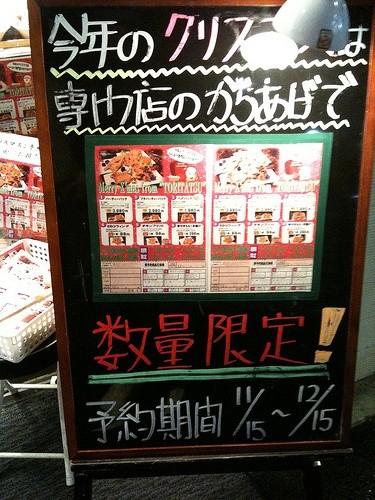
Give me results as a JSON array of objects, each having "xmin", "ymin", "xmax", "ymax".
[{"xmin": 24, "ymin": 1, "xmax": 375, "ymax": 461}]
